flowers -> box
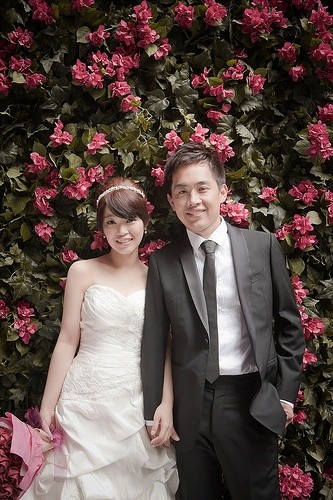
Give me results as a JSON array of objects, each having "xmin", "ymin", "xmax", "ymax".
[{"xmin": 0, "ymin": 411, "xmax": 64, "ymax": 500}]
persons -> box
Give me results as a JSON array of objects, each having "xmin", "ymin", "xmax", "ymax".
[
  {"xmin": 18, "ymin": 175, "xmax": 182, "ymax": 500},
  {"xmin": 139, "ymin": 140, "xmax": 307, "ymax": 500}
]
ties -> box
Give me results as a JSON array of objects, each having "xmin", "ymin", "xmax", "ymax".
[{"xmin": 201, "ymin": 240, "xmax": 221, "ymax": 384}]
